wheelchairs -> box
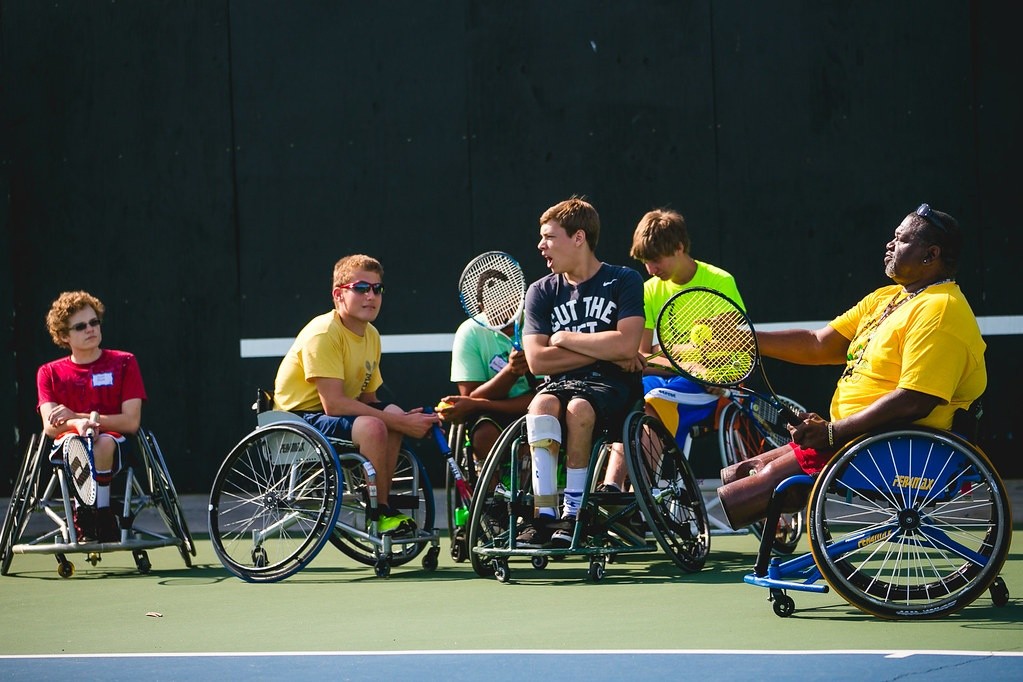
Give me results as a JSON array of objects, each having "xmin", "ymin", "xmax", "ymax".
[
  {"xmin": 0, "ymin": 425, "xmax": 197, "ymax": 578},
  {"xmin": 742, "ymin": 391, "xmax": 1014, "ymax": 624},
  {"xmin": 446, "ymin": 377, "xmax": 805, "ymax": 585},
  {"xmin": 206, "ymin": 388, "xmax": 442, "ymax": 584}
]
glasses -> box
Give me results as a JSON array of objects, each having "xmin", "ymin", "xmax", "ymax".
[
  {"xmin": 68, "ymin": 320, "xmax": 101, "ymax": 331},
  {"xmin": 917, "ymin": 203, "xmax": 950, "ymax": 234},
  {"xmin": 338, "ymin": 280, "xmax": 385, "ymax": 295}
]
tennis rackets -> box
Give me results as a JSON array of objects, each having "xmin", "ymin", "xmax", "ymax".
[
  {"xmin": 633, "ymin": 322, "xmax": 759, "ymax": 375},
  {"xmin": 656, "ymin": 286, "xmax": 807, "ymax": 428},
  {"xmin": 704, "ymin": 380, "xmax": 814, "ymax": 453},
  {"xmin": 422, "ymin": 405, "xmax": 502, "ymax": 552},
  {"xmin": 62, "ymin": 410, "xmax": 102, "ymax": 507},
  {"xmin": 458, "ymin": 250, "xmax": 538, "ymax": 388}
]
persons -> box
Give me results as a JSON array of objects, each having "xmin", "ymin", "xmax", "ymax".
[
  {"xmin": 592, "ymin": 211, "xmax": 749, "ymax": 527},
  {"xmin": 717, "ymin": 204, "xmax": 988, "ymax": 531},
  {"xmin": 270, "ymin": 255, "xmax": 446, "ymax": 542},
  {"xmin": 434, "ymin": 272, "xmax": 546, "ymax": 546},
  {"xmin": 509, "ymin": 198, "xmax": 647, "ymax": 558},
  {"xmin": 35, "ymin": 290, "xmax": 149, "ymax": 548}
]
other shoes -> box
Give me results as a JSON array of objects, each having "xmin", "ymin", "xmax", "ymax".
[
  {"xmin": 485, "ymin": 505, "xmax": 504, "ymax": 536},
  {"xmin": 597, "ymin": 484, "xmax": 619, "ymax": 493}
]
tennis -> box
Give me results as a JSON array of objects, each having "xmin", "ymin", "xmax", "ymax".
[
  {"xmin": 690, "ymin": 324, "xmax": 713, "ymax": 347},
  {"xmin": 436, "ymin": 399, "xmax": 455, "ymax": 412}
]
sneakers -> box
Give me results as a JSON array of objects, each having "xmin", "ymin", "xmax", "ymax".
[
  {"xmin": 96, "ymin": 507, "xmax": 120, "ymax": 541},
  {"xmin": 76, "ymin": 507, "xmax": 98, "ymax": 541},
  {"xmin": 515, "ymin": 522, "xmax": 552, "ymax": 548},
  {"xmin": 551, "ymin": 517, "xmax": 577, "ymax": 548},
  {"xmin": 365, "ymin": 507, "xmax": 409, "ymax": 536},
  {"xmin": 379, "ymin": 503, "xmax": 417, "ymax": 533}
]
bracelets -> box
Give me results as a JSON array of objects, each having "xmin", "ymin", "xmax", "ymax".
[{"xmin": 829, "ymin": 422, "xmax": 834, "ymax": 447}]
